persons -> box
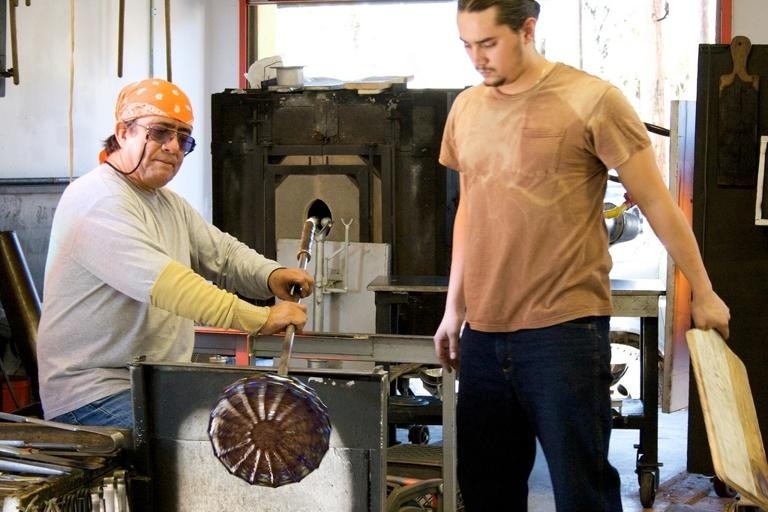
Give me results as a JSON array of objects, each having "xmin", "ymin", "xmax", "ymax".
[
  {"xmin": 35, "ymin": 79, "xmax": 316, "ymax": 434},
  {"xmin": 433, "ymin": 3, "xmax": 732, "ymax": 512}
]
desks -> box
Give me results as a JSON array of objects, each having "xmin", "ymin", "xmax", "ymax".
[{"xmin": 364, "ymin": 274, "xmax": 671, "ymax": 509}]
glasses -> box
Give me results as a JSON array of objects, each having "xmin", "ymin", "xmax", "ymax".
[{"xmin": 132, "ymin": 121, "xmax": 196, "ymax": 154}]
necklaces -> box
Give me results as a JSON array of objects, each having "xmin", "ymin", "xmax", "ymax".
[{"xmin": 535, "ymin": 59, "xmax": 548, "ymax": 84}]
